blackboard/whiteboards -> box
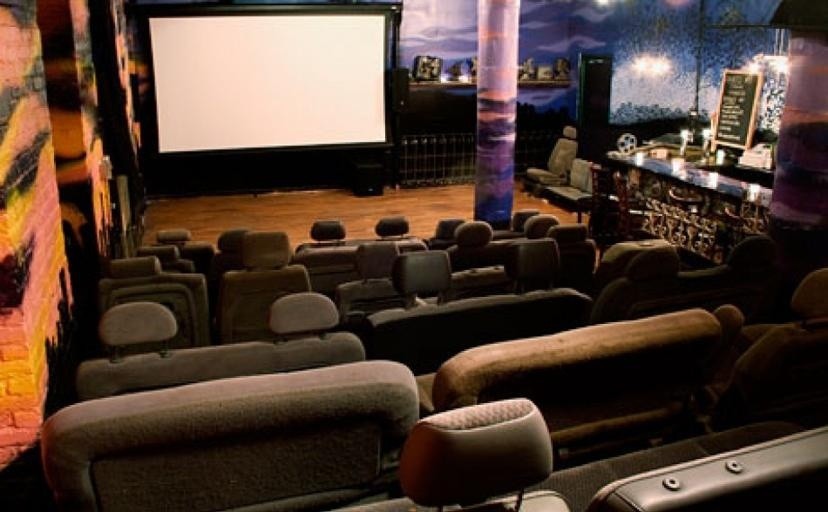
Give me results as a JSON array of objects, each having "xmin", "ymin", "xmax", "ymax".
[{"xmin": 712, "ymin": 68, "xmax": 764, "ymax": 151}]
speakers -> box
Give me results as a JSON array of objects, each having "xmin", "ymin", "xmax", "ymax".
[
  {"xmin": 577, "ymin": 52, "xmax": 613, "ymax": 129},
  {"xmin": 351, "ymin": 159, "xmax": 383, "ymax": 195},
  {"xmin": 390, "ymin": 68, "xmax": 410, "ymax": 115}
]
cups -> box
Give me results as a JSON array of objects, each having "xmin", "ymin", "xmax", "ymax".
[
  {"xmin": 749, "ymin": 184, "xmax": 760, "ymax": 201},
  {"xmin": 673, "ymin": 159, "xmax": 684, "ymax": 174},
  {"xmin": 709, "ymin": 172, "xmax": 718, "ymax": 187}
]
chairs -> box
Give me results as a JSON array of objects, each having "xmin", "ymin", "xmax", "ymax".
[
  {"xmin": 543, "ymin": 157, "xmax": 599, "ymax": 222},
  {"xmin": 37, "ymin": 207, "xmax": 827, "ymax": 511},
  {"xmin": 522, "ymin": 125, "xmax": 578, "ymax": 206}
]
